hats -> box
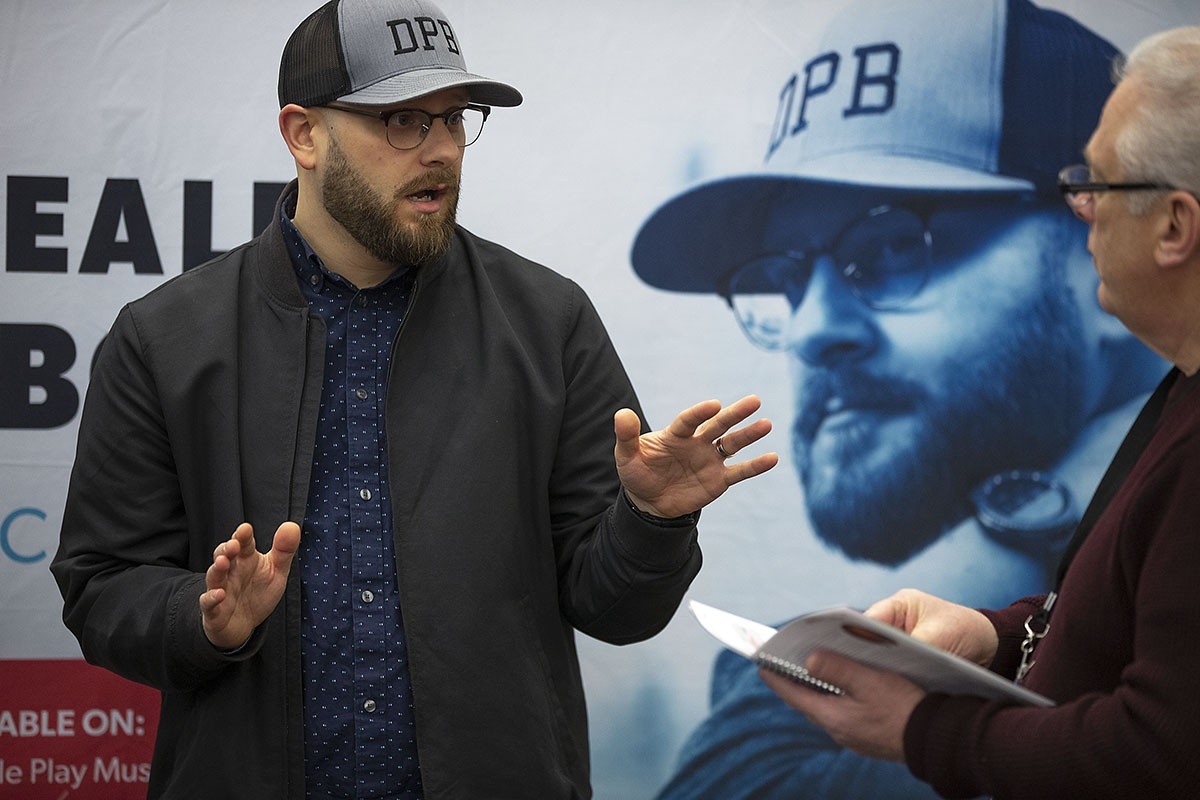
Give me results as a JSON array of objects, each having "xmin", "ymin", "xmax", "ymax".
[
  {"xmin": 278, "ymin": 0, "xmax": 523, "ymax": 108},
  {"xmin": 630, "ymin": 0, "xmax": 1131, "ymax": 294}
]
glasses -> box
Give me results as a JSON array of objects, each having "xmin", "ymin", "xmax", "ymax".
[
  {"xmin": 311, "ymin": 104, "xmax": 491, "ymax": 150},
  {"xmin": 716, "ymin": 193, "xmax": 1034, "ymax": 353},
  {"xmin": 1059, "ymin": 163, "xmax": 1179, "ymax": 209}
]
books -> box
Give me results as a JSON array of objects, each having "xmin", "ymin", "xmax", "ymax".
[{"xmin": 686, "ymin": 599, "xmax": 1054, "ymax": 711}]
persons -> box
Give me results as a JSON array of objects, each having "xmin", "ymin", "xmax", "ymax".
[
  {"xmin": 761, "ymin": 21, "xmax": 1200, "ymax": 800},
  {"xmin": 50, "ymin": 0, "xmax": 778, "ymax": 800},
  {"xmin": 630, "ymin": 0, "xmax": 1177, "ymax": 800}
]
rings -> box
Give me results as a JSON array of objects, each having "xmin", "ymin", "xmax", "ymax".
[{"xmin": 716, "ymin": 437, "xmax": 735, "ymax": 458}]
